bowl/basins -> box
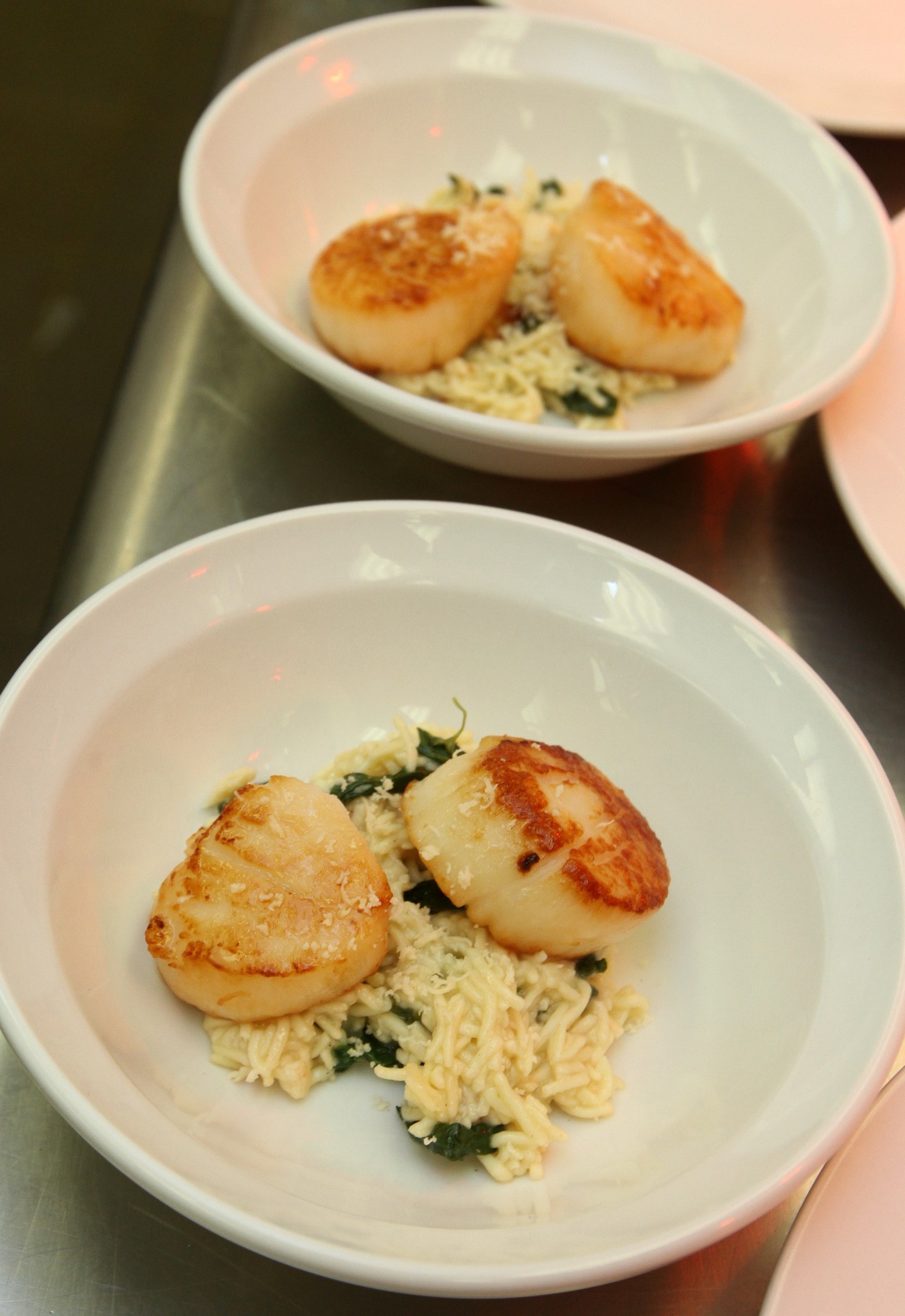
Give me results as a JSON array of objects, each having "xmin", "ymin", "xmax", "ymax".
[
  {"xmin": 176, "ymin": 9, "xmax": 900, "ymax": 487},
  {"xmin": 0, "ymin": 495, "xmax": 905, "ymax": 1300}
]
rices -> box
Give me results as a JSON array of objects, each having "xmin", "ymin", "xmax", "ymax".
[
  {"xmin": 197, "ymin": 715, "xmax": 648, "ymax": 1181},
  {"xmin": 374, "ymin": 176, "xmax": 683, "ymax": 429}
]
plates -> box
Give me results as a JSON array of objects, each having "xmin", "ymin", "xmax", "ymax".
[
  {"xmin": 755, "ymin": 1062, "xmax": 905, "ymax": 1316},
  {"xmin": 485, "ymin": 1, "xmax": 904, "ymax": 136},
  {"xmin": 818, "ymin": 210, "xmax": 905, "ymax": 607}
]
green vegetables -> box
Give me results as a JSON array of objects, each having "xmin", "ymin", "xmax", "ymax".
[
  {"xmin": 325, "ymin": 697, "xmax": 609, "ymax": 1159},
  {"xmin": 451, "ymin": 175, "xmax": 617, "ymax": 415}
]
